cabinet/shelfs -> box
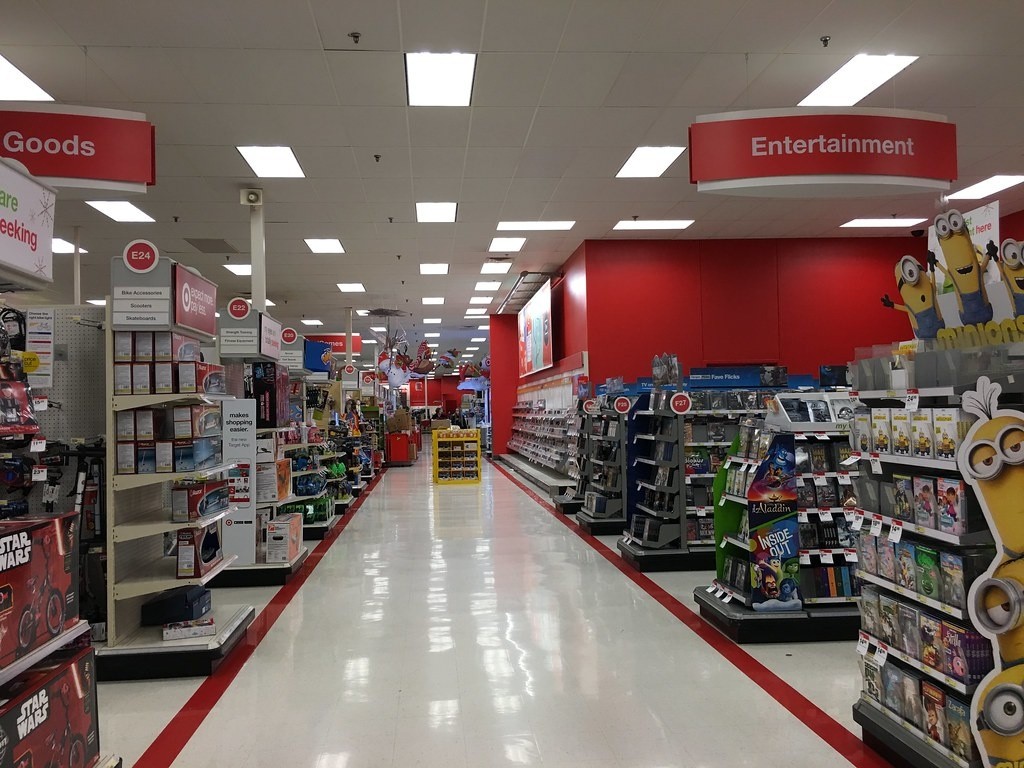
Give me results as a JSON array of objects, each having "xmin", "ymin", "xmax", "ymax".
[
  {"xmin": 0, "ymin": 154, "xmax": 123, "ymax": 768},
  {"xmin": 848, "ymin": 198, "xmax": 1024, "ymax": 768},
  {"xmin": 208, "ymin": 311, "xmax": 426, "ymax": 588},
  {"xmin": 553, "ymin": 352, "xmax": 867, "ymax": 645},
  {"xmin": 16, "ymin": 235, "xmax": 257, "ymax": 682},
  {"xmin": 431, "ymin": 428, "xmax": 482, "ymax": 484}
]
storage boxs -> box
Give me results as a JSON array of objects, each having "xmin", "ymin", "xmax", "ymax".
[
  {"xmin": 114, "ymin": 332, "xmax": 229, "ymax": 641},
  {"xmin": 265, "ymin": 513, "xmax": 303, "ymax": 565},
  {"xmin": 256, "ymin": 439, "xmax": 276, "ymax": 464},
  {"xmin": 0, "ymin": 513, "xmax": 79, "ymax": 672},
  {"xmin": 255, "ymin": 457, "xmax": 294, "ymax": 505},
  {"xmin": 853, "ymin": 407, "xmax": 976, "ymax": 759},
  {"xmin": 243, "ymin": 362, "xmax": 290, "ymax": 430},
  {"xmin": 0, "ymin": 647, "xmax": 100, "ymax": 768}
]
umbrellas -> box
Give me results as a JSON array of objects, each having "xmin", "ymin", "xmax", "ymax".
[{"xmin": 457, "ymin": 379, "xmax": 489, "ymax": 390}]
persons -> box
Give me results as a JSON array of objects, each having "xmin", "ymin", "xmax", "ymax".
[
  {"xmin": 451, "ymin": 408, "xmax": 468, "ymax": 429},
  {"xmin": 432, "ymin": 407, "xmax": 446, "ymax": 420}
]
